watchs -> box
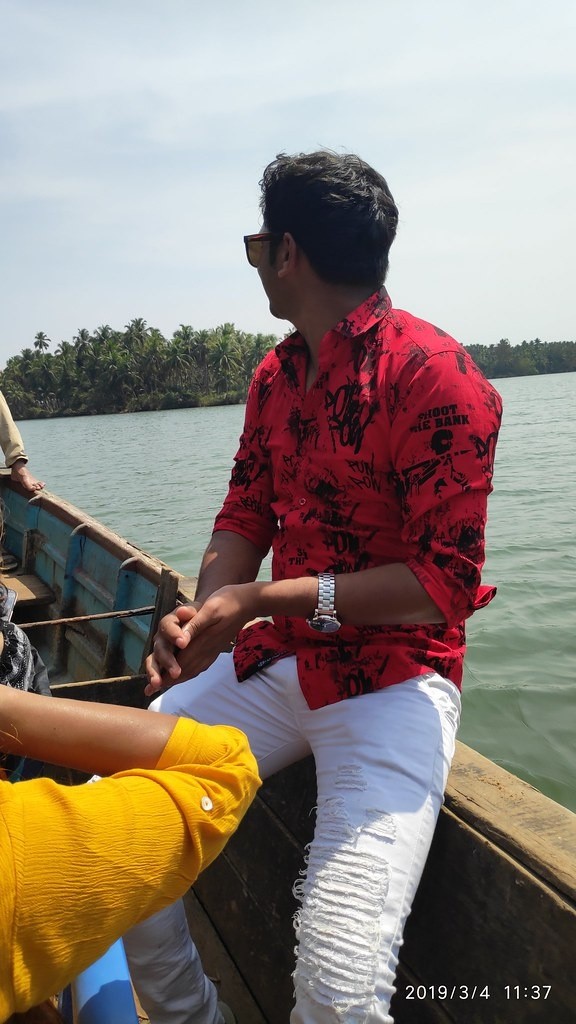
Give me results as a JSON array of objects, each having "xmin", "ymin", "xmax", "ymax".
[{"xmin": 305, "ymin": 573, "xmax": 342, "ymax": 633}]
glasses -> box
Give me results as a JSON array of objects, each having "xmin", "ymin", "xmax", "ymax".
[{"xmin": 244, "ymin": 233, "xmax": 285, "ymax": 267}]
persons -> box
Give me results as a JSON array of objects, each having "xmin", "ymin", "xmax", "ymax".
[
  {"xmin": 0, "ymin": 386, "xmax": 264, "ymax": 1023},
  {"xmin": 115, "ymin": 149, "xmax": 505, "ymax": 1024}
]
